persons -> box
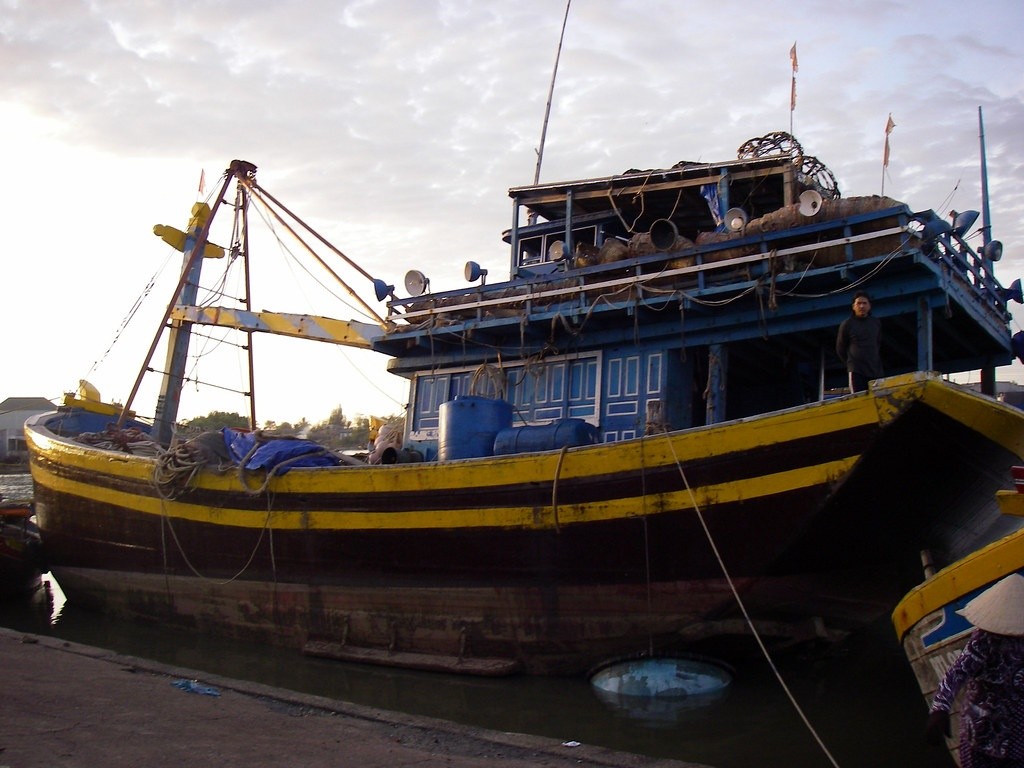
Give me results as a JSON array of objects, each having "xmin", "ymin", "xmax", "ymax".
[
  {"xmin": 920, "ymin": 571, "xmax": 1024, "ymax": 768},
  {"xmin": 836, "ymin": 291, "xmax": 888, "ymax": 396}
]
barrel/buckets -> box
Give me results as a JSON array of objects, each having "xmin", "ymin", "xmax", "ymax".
[
  {"xmin": 493, "ymin": 423, "xmax": 597, "ymax": 453},
  {"xmin": 438, "ymin": 394, "xmax": 514, "ymax": 461}
]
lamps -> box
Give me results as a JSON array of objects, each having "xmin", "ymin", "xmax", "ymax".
[
  {"xmin": 548, "ymin": 240, "xmax": 575, "ymax": 268},
  {"xmin": 464, "ymin": 261, "xmax": 488, "ymax": 287},
  {"xmin": 724, "ymin": 208, "xmax": 746, "ymax": 236},
  {"xmin": 948, "ymin": 209, "xmax": 980, "ymax": 239},
  {"xmin": 405, "ymin": 269, "xmax": 432, "ymax": 296},
  {"xmin": 373, "ymin": 278, "xmax": 396, "ymax": 303},
  {"xmin": 1003, "ymin": 278, "xmax": 1024, "ymax": 305},
  {"xmin": 977, "ymin": 240, "xmax": 1002, "ymax": 264},
  {"xmin": 799, "ymin": 190, "xmax": 823, "ymax": 223}
]
